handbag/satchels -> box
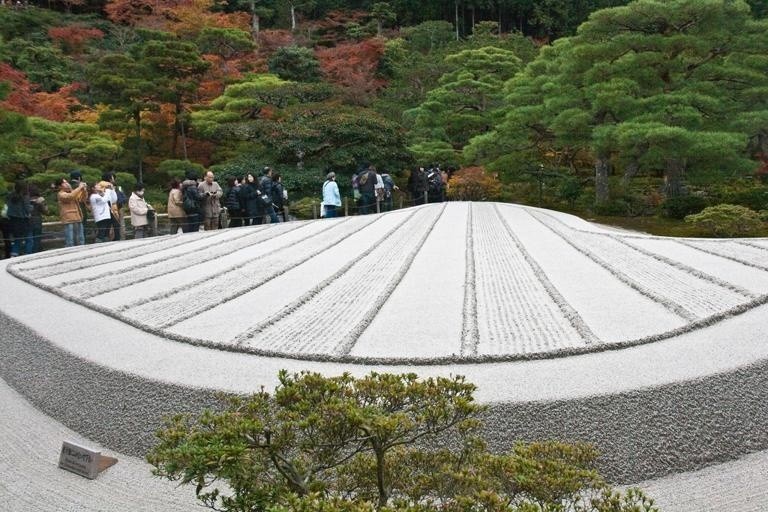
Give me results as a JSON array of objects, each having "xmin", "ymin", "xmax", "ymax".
[
  {"xmin": 320, "ymin": 202, "xmax": 325, "ymax": 217},
  {"xmin": 359, "ymin": 173, "xmax": 368, "ymax": 185},
  {"xmin": 183, "ymin": 199, "xmax": 200, "ymax": 211},
  {"xmin": 257, "ymin": 195, "xmax": 271, "ymax": 206}
]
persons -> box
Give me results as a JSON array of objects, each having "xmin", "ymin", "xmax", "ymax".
[
  {"xmin": 167, "ymin": 167, "xmax": 289, "ymax": 235},
  {"xmin": 55, "ymin": 169, "xmax": 154, "ymax": 249},
  {"xmin": 408, "ymin": 164, "xmax": 456, "ymax": 205},
  {"xmin": 6, "ymin": 178, "xmax": 47, "ymax": 256},
  {"xmin": 321, "ymin": 171, "xmax": 341, "ymax": 218},
  {"xmin": 352, "ymin": 166, "xmax": 399, "ymax": 215}
]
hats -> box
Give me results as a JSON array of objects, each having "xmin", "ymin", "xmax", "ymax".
[
  {"xmin": 71, "ymin": 171, "xmax": 82, "ymax": 181},
  {"xmin": 327, "ymin": 172, "xmax": 335, "ymax": 179}
]
